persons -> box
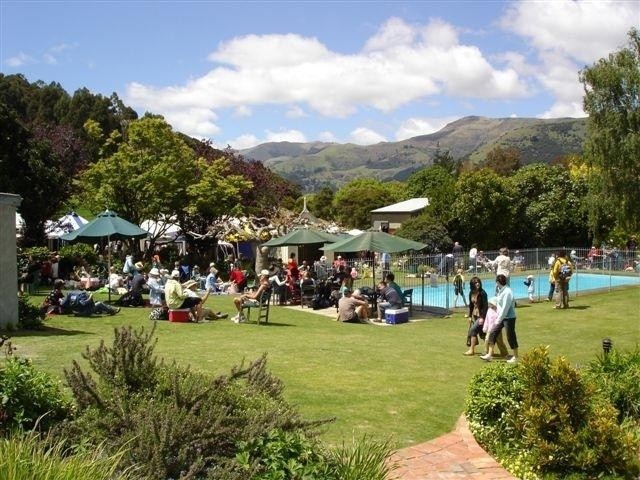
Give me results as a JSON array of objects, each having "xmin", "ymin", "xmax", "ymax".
[
  {"xmin": 478, "ymin": 296, "xmax": 509, "ymax": 358},
  {"xmin": 546, "ymin": 250, "xmax": 585, "ymax": 308},
  {"xmin": 437, "ymin": 241, "xmax": 535, "ymax": 307},
  {"xmin": 19, "ymin": 255, "xmax": 120, "ymax": 316},
  {"xmin": 587, "ymin": 234, "xmax": 638, "ymax": 272},
  {"xmin": 479, "ymin": 273, "xmax": 520, "ymax": 363},
  {"xmin": 269, "ymin": 252, "xmax": 406, "ymax": 325},
  {"xmin": 463, "ymin": 275, "xmax": 489, "ymax": 356},
  {"xmin": 92, "ymin": 240, "xmax": 271, "ymax": 324}
]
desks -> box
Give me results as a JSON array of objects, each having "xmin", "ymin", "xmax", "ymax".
[{"xmin": 361, "ymin": 291, "xmax": 381, "ymax": 312}]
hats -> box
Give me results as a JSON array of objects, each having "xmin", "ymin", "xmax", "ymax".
[
  {"xmin": 149, "ymin": 268, "xmax": 159, "ymax": 276},
  {"xmin": 257, "ymin": 269, "xmax": 269, "ymax": 276},
  {"xmin": 171, "ymin": 270, "xmax": 180, "ymax": 277}
]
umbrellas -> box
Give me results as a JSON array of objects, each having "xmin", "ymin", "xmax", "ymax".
[
  {"xmin": 46, "ymin": 212, "xmax": 90, "ymax": 240},
  {"xmin": 59, "ymin": 207, "xmax": 151, "ymax": 312},
  {"xmin": 259, "ymin": 225, "xmax": 353, "ymax": 268},
  {"xmin": 318, "ymin": 229, "xmax": 429, "ymax": 309}
]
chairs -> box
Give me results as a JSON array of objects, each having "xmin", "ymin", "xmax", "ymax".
[
  {"xmin": 403, "ymin": 289, "xmax": 413, "ymax": 318},
  {"xmin": 239, "ymin": 278, "xmax": 333, "ymax": 326}
]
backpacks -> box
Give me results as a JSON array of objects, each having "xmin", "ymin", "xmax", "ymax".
[{"xmin": 557, "ymin": 259, "xmax": 572, "ymax": 281}]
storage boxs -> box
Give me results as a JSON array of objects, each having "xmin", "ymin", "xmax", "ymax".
[
  {"xmin": 168, "ymin": 308, "xmax": 190, "ymax": 322},
  {"xmin": 385, "ymin": 307, "xmax": 409, "ymax": 324}
]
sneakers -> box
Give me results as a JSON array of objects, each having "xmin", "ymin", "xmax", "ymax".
[
  {"xmin": 110, "ymin": 308, "xmax": 121, "ymax": 315},
  {"xmin": 216, "ymin": 312, "xmax": 228, "ymax": 318},
  {"xmin": 362, "ymin": 319, "xmax": 370, "ymax": 324},
  {"xmin": 462, "ymin": 350, "xmax": 518, "ymax": 363},
  {"xmin": 231, "ymin": 317, "xmax": 245, "ymax": 323},
  {"xmin": 198, "ymin": 318, "xmax": 209, "ymax": 322},
  {"xmin": 373, "ymin": 319, "xmax": 382, "ymax": 323},
  {"xmin": 553, "ymin": 305, "xmax": 568, "ymax": 309}
]
